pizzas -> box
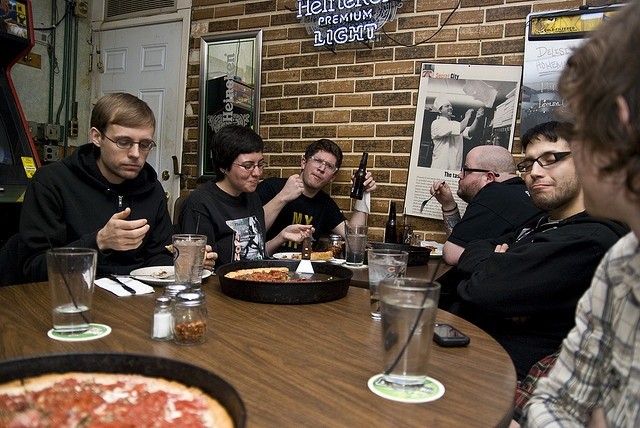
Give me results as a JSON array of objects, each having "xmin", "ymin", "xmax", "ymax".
[
  {"xmin": 2, "ymin": 372, "xmax": 235, "ymax": 427},
  {"xmin": 226, "ymin": 266, "xmax": 326, "ymax": 282},
  {"xmin": 282, "ymin": 249, "xmax": 337, "ymax": 261},
  {"xmin": 422, "ymin": 243, "xmax": 438, "ymax": 253}
]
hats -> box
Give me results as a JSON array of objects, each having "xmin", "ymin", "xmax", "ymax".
[{"xmin": 434, "ymin": 96, "xmax": 451, "ymax": 111}]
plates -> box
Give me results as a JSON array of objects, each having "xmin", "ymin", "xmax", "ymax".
[
  {"xmin": 131, "ymin": 267, "xmax": 211, "ymax": 289},
  {"xmin": 274, "ymin": 252, "xmax": 347, "ymax": 266},
  {"xmin": 416, "ymin": 243, "xmax": 445, "ymax": 258}
]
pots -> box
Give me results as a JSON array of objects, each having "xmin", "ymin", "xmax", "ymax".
[
  {"xmin": 343, "ymin": 241, "xmax": 432, "ymax": 267},
  {"xmin": 216, "ymin": 261, "xmax": 353, "ymax": 304},
  {"xmin": 0, "ymin": 351, "xmax": 247, "ymax": 428}
]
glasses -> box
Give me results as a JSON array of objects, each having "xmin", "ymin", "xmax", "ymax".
[
  {"xmin": 552, "ymin": 106, "xmax": 590, "ymax": 142},
  {"xmin": 233, "ymin": 161, "xmax": 265, "ymax": 171},
  {"xmin": 103, "ymin": 133, "xmax": 157, "ymax": 151},
  {"xmin": 517, "ymin": 152, "xmax": 573, "ymax": 172},
  {"xmin": 309, "ymin": 156, "xmax": 337, "ymax": 174},
  {"xmin": 461, "ymin": 167, "xmax": 499, "ymax": 179}
]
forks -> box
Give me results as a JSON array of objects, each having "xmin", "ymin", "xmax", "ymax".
[{"xmin": 420, "ymin": 181, "xmax": 448, "ymax": 213}]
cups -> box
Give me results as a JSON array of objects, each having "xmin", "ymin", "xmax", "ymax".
[
  {"xmin": 171, "ymin": 233, "xmax": 207, "ymax": 291},
  {"xmin": 380, "ymin": 277, "xmax": 440, "ymax": 387},
  {"xmin": 368, "ymin": 247, "xmax": 407, "ymax": 319},
  {"xmin": 343, "ymin": 224, "xmax": 368, "ymax": 266},
  {"xmin": 45, "ymin": 247, "xmax": 97, "ymax": 336}
]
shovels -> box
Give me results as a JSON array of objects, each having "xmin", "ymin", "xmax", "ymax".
[{"xmin": 292, "ymin": 237, "xmax": 316, "ymax": 280}]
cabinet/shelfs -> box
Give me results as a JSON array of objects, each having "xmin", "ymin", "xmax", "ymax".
[{"xmin": 1, "ymin": 1, "xmax": 42, "ymax": 249}]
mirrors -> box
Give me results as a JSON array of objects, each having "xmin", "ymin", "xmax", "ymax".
[{"xmin": 198, "ymin": 29, "xmax": 263, "ymax": 183}]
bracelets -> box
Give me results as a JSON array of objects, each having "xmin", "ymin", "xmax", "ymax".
[{"xmin": 441, "ymin": 204, "xmax": 457, "ymax": 213}]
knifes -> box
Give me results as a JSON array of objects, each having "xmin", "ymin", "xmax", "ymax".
[{"xmin": 105, "ymin": 273, "xmax": 136, "ymax": 296}]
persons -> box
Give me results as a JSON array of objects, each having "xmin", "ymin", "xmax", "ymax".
[
  {"xmin": 430, "ymin": 145, "xmax": 544, "ymax": 265},
  {"xmin": 177, "ymin": 126, "xmax": 317, "ymax": 273},
  {"xmin": 432, "ymin": 96, "xmax": 484, "ymax": 170},
  {"xmin": 256, "ymin": 140, "xmax": 377, "ymax": 253},
  {"xmin": 515, "ymin": 0, "xmax": 640, "ymax": 428},
  {"xmin": 448, "ymin": 122, "xmax": 630, "ymax": 381},
  {"xmin": 18, "ymin": 92, "xmax": 219, "ymax": 282}
]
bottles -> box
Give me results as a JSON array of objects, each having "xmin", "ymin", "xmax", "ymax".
[
  {"xmin": 349, "ymin": 152, "xmax": 368, "ymax": 200},
  {"xmin": 328, "ymin": 235, "xmax": 341, "ymax": 259},
  {"xmin": 405, "ymin": 229, "xmax": 414, "ymax": 245},
  {"xmin": 171, "ymin": 288, "xmax": 207, "ymax": 347},
  {"xmin": 384, "ymin": 200, "xmax": 398, "ymax": 243},
  {"xmin": 150, "ymin": 296, "xmax": 173, "ymax": 341},
  {"xmin": 412, "ymin": 234, "xmax": 422, "ymax": 247},
  {"xmin": 165, "ymin": 284, "xmax": 188, "ymax": 304}
]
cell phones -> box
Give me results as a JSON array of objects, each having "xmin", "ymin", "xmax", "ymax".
[{"xmin": 433, "ymin": 322, "xmax": 470, "ymax": 348}]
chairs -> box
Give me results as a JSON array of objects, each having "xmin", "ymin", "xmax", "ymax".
[
  {"xmin": 173, "ymin": 196, "xmax": 188, "ymax": 224},
  {"xmin": 0, "ymin": 233, "xmax": 20, "ymax": 287}
]
